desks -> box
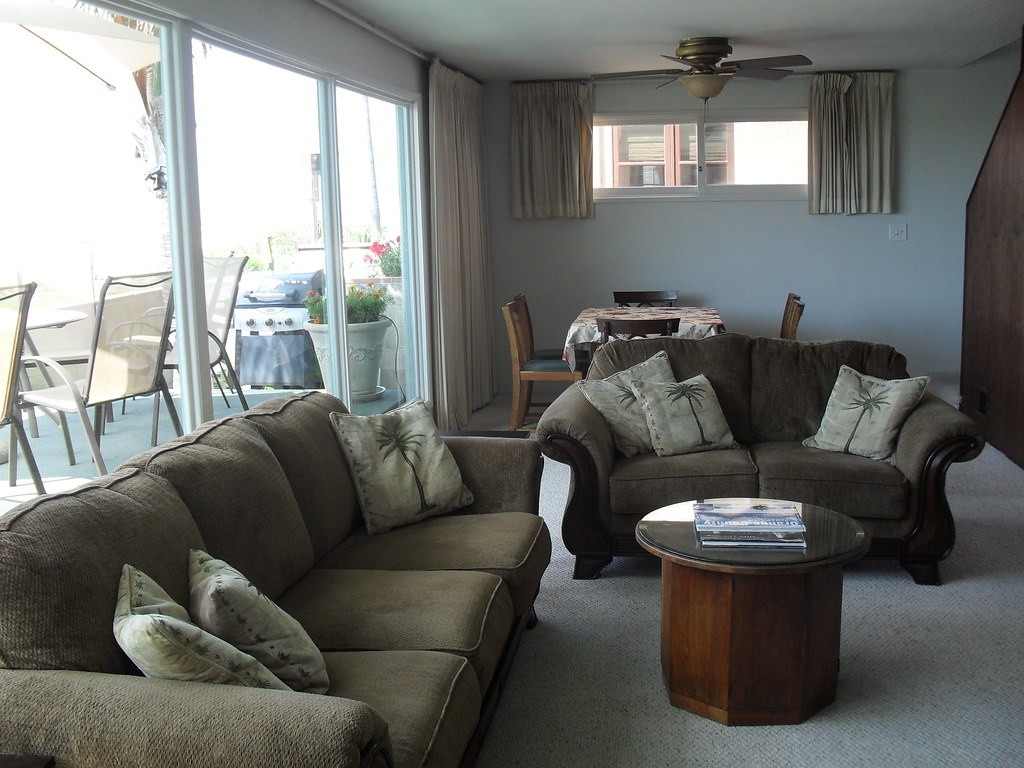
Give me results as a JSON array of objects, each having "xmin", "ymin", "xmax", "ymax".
[
  {"xmin": 562, "ymin": 307, "xmax": 726, "ymax": 373},
  {"xmin": 19, "ymin": 310, "xmax": 88, "ymax": 438}
]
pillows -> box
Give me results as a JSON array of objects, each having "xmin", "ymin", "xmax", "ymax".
[
  {"xmin": 113, "ymin": 547, "xmax": 329, "ymax": 691},
  {"xmin": 329, "ymin": 396, "xmax": 474, "ymax": 533},
  {"xmin": 802, "ymin": 364, "xmax": 930, "ymax": 466},
  {"xmin": 574, "ymin": 350, "xmax": 740, "ymax": 456}
]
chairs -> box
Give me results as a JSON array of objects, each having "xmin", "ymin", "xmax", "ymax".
[
  {"xmin": 613, "ymin": 290, "xmax": 678, "ymax": 307},
  {"xmin": 0, "ymin": 256, "xmax": 248, "ymax": 496},
  {"xmin": 501, "ymin": 292, "xmax": 590, "ymax": 432},
  {"xmin": 596, "ymin": 318, "xmax": 681, "ymax": 345},
  {"xmin": 779, "ymin": 293, "xmax": 805, "ymax": 341}
]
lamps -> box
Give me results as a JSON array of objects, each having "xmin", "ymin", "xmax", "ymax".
[{"xmin": 677, "ymin": 75, "xmax": 731, "ymax": 106}]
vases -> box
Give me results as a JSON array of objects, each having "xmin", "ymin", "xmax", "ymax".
[{"xmin": 303, "ymin": 319, "xmax": 392, "ymax": 395}]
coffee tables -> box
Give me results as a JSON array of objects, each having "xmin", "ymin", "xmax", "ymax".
[{"xmin": 635, "ymin": 498, "xmax": 871, "ymax": 726}]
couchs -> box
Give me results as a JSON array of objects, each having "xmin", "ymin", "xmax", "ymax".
[
  {"xmin": 536, "ymin": 333, "xmax": 987, "ymax": 588},
  {"xmin": 0, "ymin": 391, "xmax": 552, "ymax": 768}
]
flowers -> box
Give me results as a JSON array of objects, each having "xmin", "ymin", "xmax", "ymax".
[{"xmin": 304, "ymin": 281, "xmax": 387, "ymax": 324}]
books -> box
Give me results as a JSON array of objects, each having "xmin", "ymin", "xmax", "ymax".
[{"xmin": 693, "ymin": 502, "xmax": 807, "ymax": 548}]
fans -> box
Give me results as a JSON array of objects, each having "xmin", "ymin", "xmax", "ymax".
[{"xmin": 590, "ymin": 37, "xmax": 812, "ymax": 90}]
